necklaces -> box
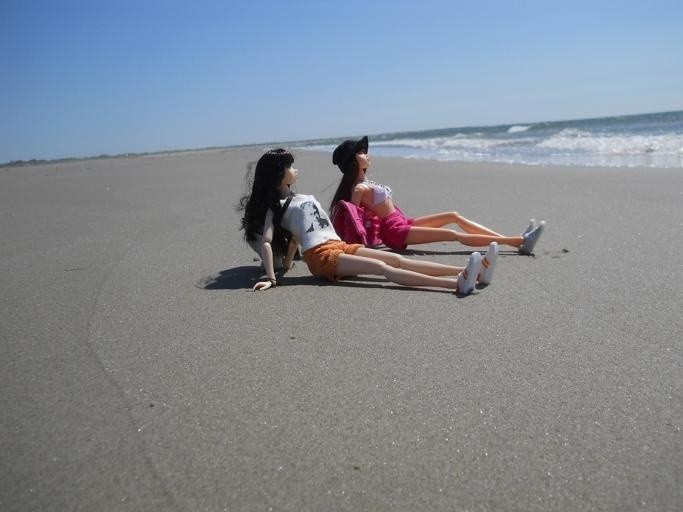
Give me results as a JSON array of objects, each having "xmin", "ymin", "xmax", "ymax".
[{"xmin": 358, "ymin": 177, "xmax": 392, "ymax": 194}]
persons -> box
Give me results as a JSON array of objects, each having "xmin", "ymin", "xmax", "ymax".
[
  {"xmin": 237, "ymin": 148, "xmax": 498, "ymax": 296},
  {"xmin": 325, "ymin": 134, "xmax": 546, "ymax": 255}
]
